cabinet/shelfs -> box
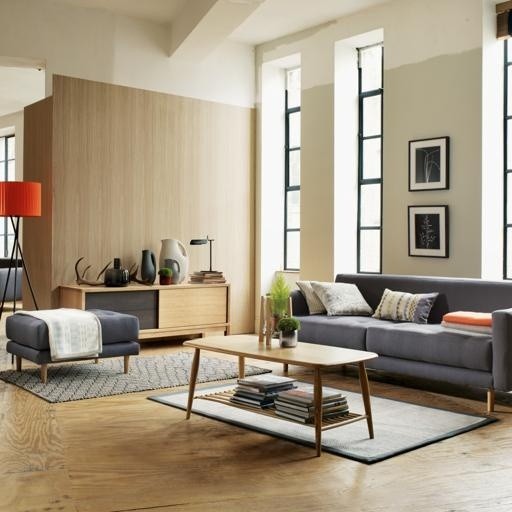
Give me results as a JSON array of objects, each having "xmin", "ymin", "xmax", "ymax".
[{"xmin": 59, "ymin": 283, "xmax": 231, "ymax": 340}]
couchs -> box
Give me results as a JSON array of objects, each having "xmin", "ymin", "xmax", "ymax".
[{"xmin": 290, "ymin": 274, "xmax": 512, "ymax": 412}]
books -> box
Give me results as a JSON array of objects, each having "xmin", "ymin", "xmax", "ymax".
[
  {"xmin": 229, "ymin": 373, "xmax": 349, "ymax": 424},
  {"xmin": 187, "ymin": 270, "xmax": 225, "ymax": 283}
]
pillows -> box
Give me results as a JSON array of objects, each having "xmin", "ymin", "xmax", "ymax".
[
  {"xmin": 311, "ymin": 281, "xmax": 374, "ymax": 317},
  {"xmin": 296, "ymin": 281, "xmax": 332, "ymax": 315},
  {"xmin": 372, "ymin": 289, "xmax": 440, "ymax": 324}
]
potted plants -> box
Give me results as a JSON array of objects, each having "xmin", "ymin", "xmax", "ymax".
[{"xmin": 268, "ymin": 270, "xmax": 301, "ymax": 348}]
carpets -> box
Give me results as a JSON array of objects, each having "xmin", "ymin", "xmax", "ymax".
[
  {"xmin": 147, "ymin": 373, "xmax": 498, "ymax": 465},
  {"xmin": 0, "ymin": 350, "xmax": 272, "ymax": 404}
]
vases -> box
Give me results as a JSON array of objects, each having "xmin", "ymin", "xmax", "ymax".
[{"xmin": 104, "ymin": 239, "xmax": 190, "ymax": 287}]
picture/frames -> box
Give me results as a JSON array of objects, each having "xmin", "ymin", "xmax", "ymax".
[
  {"xmin": 408, "ymin": 136, "xmax": 449, "ymax": 192},
  {"xmin": 407, "ymin": 205, "xmax": 449, "ymax": 258}
]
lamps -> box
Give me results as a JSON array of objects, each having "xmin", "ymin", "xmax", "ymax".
[
  {"xmin": 190, "ymin": 235, "xmax": 218, "ymax": 272},
  {"xmin": 0, "ymin": 181, "xmax": 41, "ymax": 365}
]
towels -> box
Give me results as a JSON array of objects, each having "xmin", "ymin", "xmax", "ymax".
[
  {"xmin": 14, "ymin": 308, "xmax": 103, "ymax": 361},
  {"xmin": 441, "ymin": 311, "xmax": 492, "ymax": 334}
]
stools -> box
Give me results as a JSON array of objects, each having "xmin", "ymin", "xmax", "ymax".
[{"xmin": 5, "ymin": 308, "xmax": 140, "ymax": 384}]
